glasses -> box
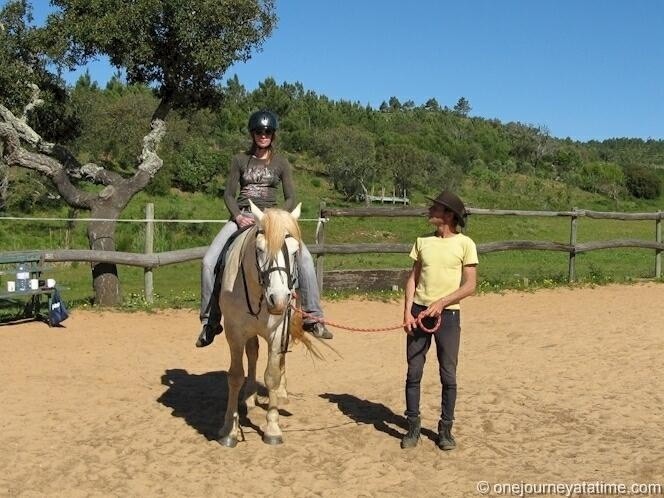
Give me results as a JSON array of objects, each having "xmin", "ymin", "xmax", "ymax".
[{"xmin": 251, "ymin": 129, "xmax": 274, "ymax": 135}]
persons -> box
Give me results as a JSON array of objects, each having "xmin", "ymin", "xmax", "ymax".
[
  {"xmin": 400, "ymin": 190, "xmax": 479, "ymax": 451},
  {"xmin": 195, "ymin": 109, "xmax": 334, "ymax": 349}
]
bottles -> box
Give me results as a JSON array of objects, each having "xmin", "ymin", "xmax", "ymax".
[{"xmin": 15, "ymin": 261, "xmax": 30, "ymax": 294}]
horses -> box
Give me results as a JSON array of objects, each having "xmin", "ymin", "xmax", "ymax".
[{"xmin": 215, "ymin": 198, "xmax": 344, "ymax": 448}]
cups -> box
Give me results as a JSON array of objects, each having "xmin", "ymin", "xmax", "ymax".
[
  {"xmin": 30, "ymin": 279, "xmax": 39, "ymax": 290},
  {"xmin": 7, "ymin": 280, "xmax": 16, "ymax": 293},
  {"xmin": 47, "ymin": 278, "xmax": 57, "ymax": 289}
]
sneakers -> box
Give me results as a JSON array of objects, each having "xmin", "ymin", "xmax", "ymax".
[
  {"xmin": 301, "ymin": 319, "xmax": 333, "ymax": 339},
  {"xmin": 195, "ymin": 322, "xmax": 224, "ymax": 347}
]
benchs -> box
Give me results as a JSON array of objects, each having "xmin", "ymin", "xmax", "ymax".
[{"xmin": 0, "ymin": 250, "xmax": 71, "ymax": 325}]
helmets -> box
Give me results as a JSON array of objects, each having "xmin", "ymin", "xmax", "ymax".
[{"xmin": 247, "ymin": 110, "xmax": 278, "ymax": 132}]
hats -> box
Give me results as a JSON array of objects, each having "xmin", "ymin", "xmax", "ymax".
[{"xmin": 423, "ymin": 190, "xmax": 468, "ymax": 228}]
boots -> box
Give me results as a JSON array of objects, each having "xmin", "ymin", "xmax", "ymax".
[
  {"xmin": 437, "ymin": 418, "xmax": 457, "ymax": 450},
  {"xmin": 400, "ymin": 415, "xmax": 423, "ymax": 448}
]
